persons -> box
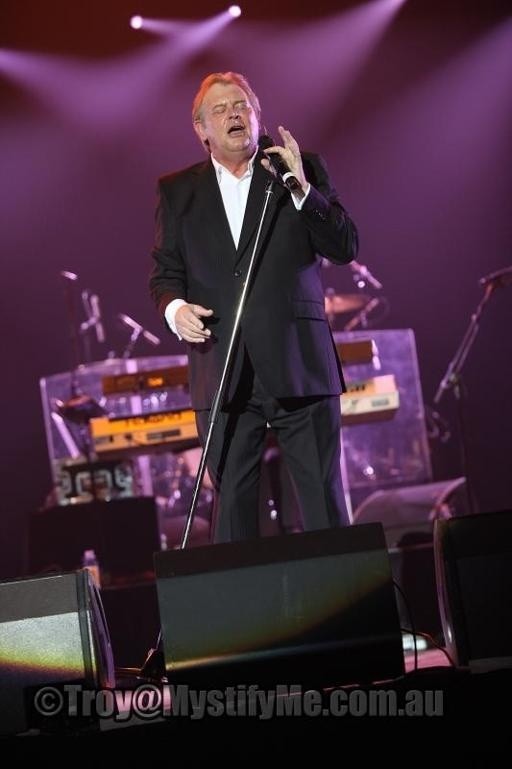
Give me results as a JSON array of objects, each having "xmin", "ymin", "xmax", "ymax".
[{"xmin": 147, "ymin": 70, "xmax": 359, "ymax": 545}]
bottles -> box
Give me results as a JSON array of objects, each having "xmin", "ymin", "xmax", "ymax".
[{"xmin": 81, "ymin": 550, "xmax": 101, "ymax": 590}]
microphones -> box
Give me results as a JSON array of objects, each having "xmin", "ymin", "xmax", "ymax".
[
  {"xmin": 257, "ymin": 135, "xmax": 302, "ymax": 193},
  {"xmin": 480, "ymin": 262, "xmax": 512, "ymax": 283},
  {"xmin": 121, "ymin": 311, "xmax": 158, "ymax": 345},
  {"xmin": 343, "ymin": 258, "xmax": 384, "ymax": 291}
]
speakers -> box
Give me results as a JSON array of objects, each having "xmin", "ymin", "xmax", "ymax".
[
  {"xmin": 432, "ymin": 507, "xmax": 512, "ymax": 667},
  {"xmin": 155, "ymin": 521, "xmax": 402, "ymax": 701},
  {"xmin": 0, "ymin": 566, "xmax": 118, "ymax": 727}
]
